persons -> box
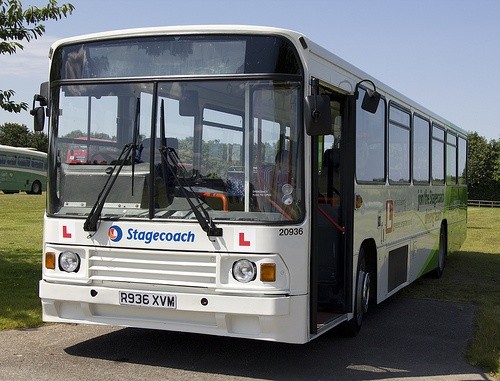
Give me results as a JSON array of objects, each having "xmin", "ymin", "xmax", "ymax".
[{"xmin": 262, "ymin": 149, "xmax": 293, "ymax": 213}]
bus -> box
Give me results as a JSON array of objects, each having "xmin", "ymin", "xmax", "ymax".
[
  {"xmin": 0, "ymin": 145, "xmax": 48, "ymax": 194},
  {"xmin": 67, "ymin": 137, "xmax": 122, "ymax": 166},
  {"xmin": 30, "ymin": 24, "xmax": 468, "ymax": 344}
]
